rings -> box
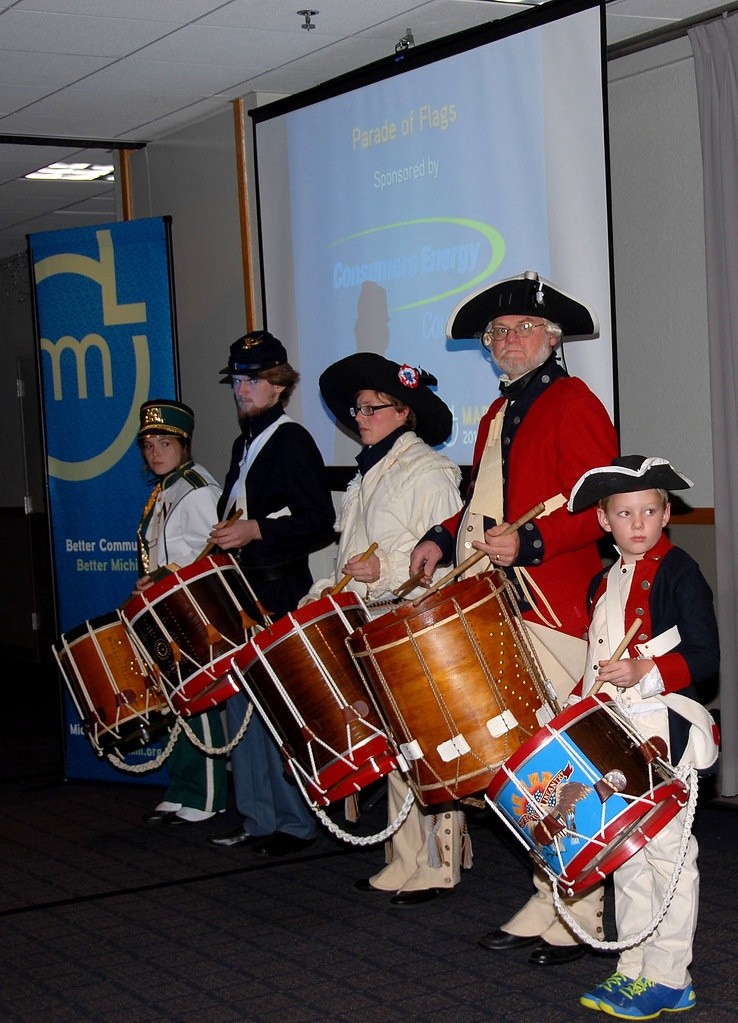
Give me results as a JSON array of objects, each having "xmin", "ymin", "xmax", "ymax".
[{"xmin": 496, "ymin": 555, "xmax": 500, "ymax": 561}]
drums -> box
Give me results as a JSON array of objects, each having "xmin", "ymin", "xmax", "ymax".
[
  {"xmin": 120, "ymin": 554, "xmax": 278, "ymax": 713},
  {"xmin": 46, "ymin": 606, "xmax": 181, "ymax": 757},
  {"xmin": 479, "ymin": 690, "xmax": 690, "ymax": 900},
  {"xmin": 228, "ymin": 586, "xmax": 399, "ymax": 807},
  {"xmin": 345, "ymin": 571, "xmax": 556, "ymax": 813}
]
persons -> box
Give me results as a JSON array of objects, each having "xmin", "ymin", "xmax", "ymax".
[
  {"xmin": 207, "ymin": 331, "xmax": 342, "ymax": 856},
  {"xmin": 134, "ymin": 400, "xmax": 225, "ymax": 833},
  {"xmin": 568, "ymin": 455, "xmax": 721, "ymax": 1019},
  {"xmin": 409, "ymin": 268, "xmax": 621, "ymax": 947},
  {"xmin": 299, "ymin": 349, "xmax": 476, "ymax": 893}
]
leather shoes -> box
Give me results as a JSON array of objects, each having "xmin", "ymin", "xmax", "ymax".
[
  {"xmin": 390, "ymin": 882, "xmax": 463, "ymax": 908},
  {"xmin": 528, "ymin": 941, "xmax": 587, "ymax": 966},
  {"xmin": 252, "ymin": 832, "xmax": 316, "ymax": 858},
  {"xmin": 164, "ymin": 813, "xmax": 227, "ymax": 828},
  {"xmin": 139, "ymin": 808, "xmax": 177, "ymax": 823},
  {"xmin": 477, "ymin": 927, "xmax": 544, "ymax": 950},
  {"xmin": 206, "ymin": 827, "xmax": 262, "ymax": 849},
  {"xmin": 352, "ymin": 878, "xmax": 392, "ymax": 896}
]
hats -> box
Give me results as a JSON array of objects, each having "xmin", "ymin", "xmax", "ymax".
[
  {"xmin": 320, "ymin": 352, "xmax": 453, "ymax": 447},
  {"xmin": 445, "ymin": 271, "xmax": 600, "ymax": 340},
  {"xmin": 136, "ymin": 398, "xmax": 194, "ymax": 441},
  {"xmin": 218, "ymin": 331, "xmax": 287, "ymax": 376},
  {"xmin": 567, "ymin": 455, "xmax": 691, "ymax": 516}
]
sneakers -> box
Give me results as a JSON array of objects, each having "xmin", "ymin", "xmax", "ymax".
[
  {"xmin": 580, "ymin": 972, "xmax": 636, "ymax": 1012},
  {"xmin": 600, "ymin": 976, "xmax": 695, "ymax": 1020}
]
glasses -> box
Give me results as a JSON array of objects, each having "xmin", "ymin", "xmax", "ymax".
[
  {"xmin": 486, "ymin": 322, "xmax": 549, "ymax": 342},
  {"xmin": 349, "ymin": 401, "xmax": 398, "ymax": 418}
]
